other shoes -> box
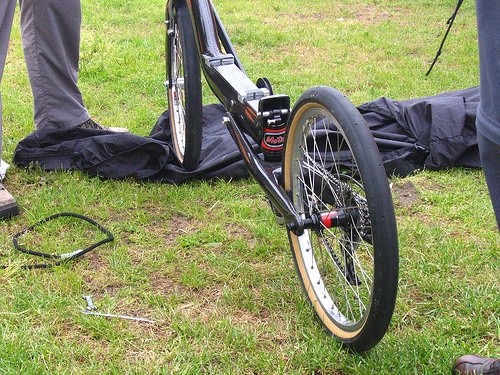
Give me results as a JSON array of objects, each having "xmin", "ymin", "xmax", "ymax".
[
  {"xmin": 69, "ymin": 118, "xmax": 132, "ymax": 138},
  {"xmin": 1, "ymin": 179, "xmax": 20, "ymax": 220}
]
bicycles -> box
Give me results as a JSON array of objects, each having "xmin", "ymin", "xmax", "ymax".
[{"xmin": 158, "ymin": 0, "xmax": 399, "ymax": 350}]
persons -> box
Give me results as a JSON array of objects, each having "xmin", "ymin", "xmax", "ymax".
[
  {"xmin": 451, "ymin": 0, "xmax": 500, "ymax": 375},
  {"xmin": 0, "ymin": 0, "xmax": 129, "ymax": 218}
]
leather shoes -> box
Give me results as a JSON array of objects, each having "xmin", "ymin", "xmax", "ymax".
[{"xmin": 451, "ymin": 353, "xmax": 500, "ymax": 374}]
